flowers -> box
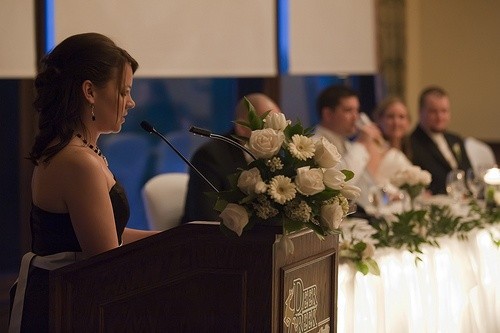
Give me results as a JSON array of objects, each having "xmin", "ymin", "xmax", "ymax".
[{"xmin": 214, "ymin": 103, "xmax": 500, "ymax": 275}]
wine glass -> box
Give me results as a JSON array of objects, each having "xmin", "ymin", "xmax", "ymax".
[
  {"xmin": 466, "ymin": 167, "xmax": 484, "ymax": 205},
  {"xmin": 445, "ymin": 170, "xmax": 465, "ymax": 211}
]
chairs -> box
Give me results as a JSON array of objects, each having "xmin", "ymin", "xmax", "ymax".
[{"xmin": 142, "ymin": 173, "xmax": 189, "ymax": 230}]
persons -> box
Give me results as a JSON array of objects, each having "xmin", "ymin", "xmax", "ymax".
[
  {"xmin": 177, "ymin": 93, "xmax": 283, "ymax": 227},
  {"xmin": 9, "ymin": 33, "xmax": 163, "ymax": 333},
  {"xmin": 305, "ymin": 83, "xmax": 476, "ymax": 213}
]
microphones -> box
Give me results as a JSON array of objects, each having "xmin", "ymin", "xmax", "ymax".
[
  {"xmin": 189, "ymin": 125, "xmax": 257, "ymax": 161},
  {"xmin": 140, "ymin": 120, "xmax": 219, "ymax": 193}
]
